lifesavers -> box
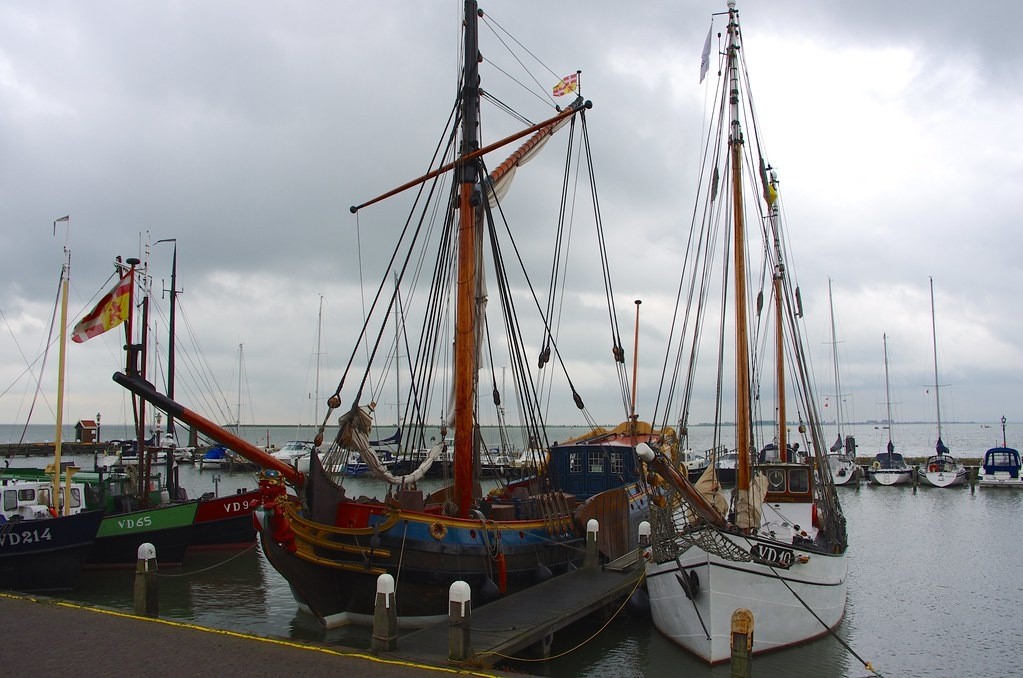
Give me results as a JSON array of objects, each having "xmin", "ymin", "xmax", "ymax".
[
  {"xmin": 873, "ymin": 461, "xmax": 881, "ymax": 469},
  {"xmin": 930, "ymin": 464, "xmax": 939, "ymax": 473}
]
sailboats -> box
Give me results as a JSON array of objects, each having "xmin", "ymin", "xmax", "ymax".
[
  {"xmin": 255, "ymin": 1, "xmax": 690, "ymax": 615},
  {"xmin": 866, "ymin": 332, "xmax": 915, "ymax": 486},
  {"xmin": 193, "ymin": 343, "xmax": 279, "ymax": 471},
  {"xmin": 1, "ymin": 215, "xmax": 164, "ymax": 512},
  {"xmin": 639, "ymin": 0, "xmax": 847, "ymax": 666},
  {"xmin": 814, "ymin": 279, "xmax": 865, "ymax": 486},
  {"xmin": 918, "ymin": 275, "xmax": 966, "ymax": 488}
]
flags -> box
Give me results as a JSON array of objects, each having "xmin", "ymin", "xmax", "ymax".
[
  {"xmin": 71, "ymin": 270, "xmax": 133, "ymax": 343},
  {"xmin": 699, "ymin": 27, "xmax": 710, "ymax": 85},
  {"xmin": 553, "ymin": 73, "xmax": 578, "ymax": 97}
]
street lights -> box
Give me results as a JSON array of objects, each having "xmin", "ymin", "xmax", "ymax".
[
  {"xmin": 270, "ymin": 294, "xmax": 359, "ymax": 475},
  {"xmin": 95, "ymin": 412, "xmax": 102, "ymax": 443},
  {"xmin": 1001, "ymin": 415, "xmax": 1007, "ymax": 448},
  {"xmin": 155, "ymin": 412, "xmax": 162, "ymax": 446},
  {"xmin": 174, "ymin": 446, "xmax": 196, "ymax": 463}
]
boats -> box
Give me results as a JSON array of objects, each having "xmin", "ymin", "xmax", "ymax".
[
  {"xmin": 875, "ymin": 426, "xmax": 879, "ymax": 429},
  {"xmin": 976, "ymin": 448, "xmax": 1023, "ymax": 486},
  {"xmin": 756, "ymin": 443, "xmax": 807, "ymax": 464},
  {"xmin": 883, "ymin": 426, "xmax": 889, "ymax": 429},
  {"xmin": 95, "ymin": 437, "xmax": 179, "ymax": 468},
  {"xmin": 514, "ymin": 449, "xmax": 547, "ymax": 468},
  {"xmin": 340, "ymin": 450, "xmax": 399, "ymax": 480},
  {"xmin": 480, "ymin": 447, "xmax": 512, "ymax": 467},
  {"xmin": 680, "ymin": 429, "xmax": 710, "ymax": 484},
  {"xmin": 1, "ymin": 232, "xmax": 288, "ymax": 569},
  {"xmin": 435, "ymin": 438, "xmax": 456, "ymax": 462}
]
